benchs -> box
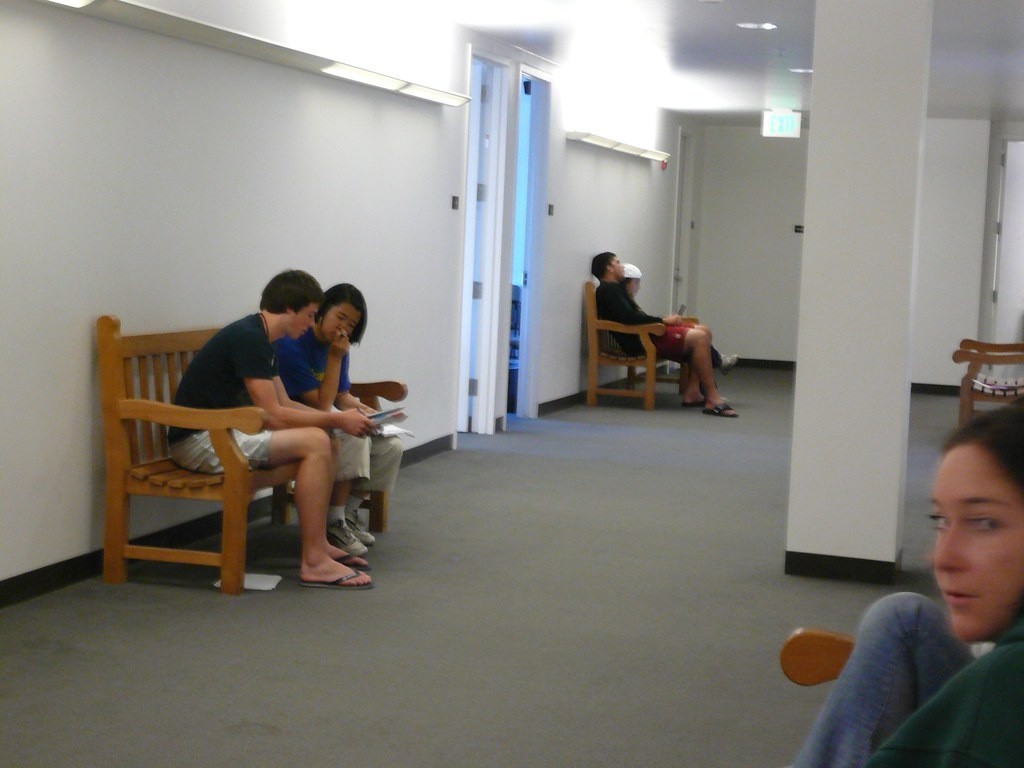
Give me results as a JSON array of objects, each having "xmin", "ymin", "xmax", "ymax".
[
  {"xmin": 584, "ymin": 282, "xmax": 699, "ymax": 410},
  {"xmin": 96, "ymin": 314, "xmax": 408, "ymax": 595},
  {"xmin": 954, "ymin": 339, "xmax": 1024, "ymax": 427}
]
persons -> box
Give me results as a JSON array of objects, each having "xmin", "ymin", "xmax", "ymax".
[
  {"xmin": 791, "ymin": 396, "xmax": 1024, "ymax": 768},
  {"xmin": 591, "ymin": 251, "xmax": 738, "ymax": 418},
  {"xmin": 166, "ymin": 269, "xmax": 403, "ymax": 590}
]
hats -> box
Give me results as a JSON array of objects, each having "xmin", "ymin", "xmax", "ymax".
[{"xmin": 621, "ymin": 263, "xmax": 642, "ymax": 281}]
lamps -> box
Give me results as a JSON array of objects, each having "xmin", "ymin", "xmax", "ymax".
[
  {"xmin": 42, "ymin": 0, "xmax": 471, "ymax": 108},
  {"xmin": 567, "ymin": 132, "xmax": 671, "ymax": 161}
]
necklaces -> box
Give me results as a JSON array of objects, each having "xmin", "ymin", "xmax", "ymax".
[{"xmin": 260, "ymin": 312, "xmax": 276, "ymax": 366}]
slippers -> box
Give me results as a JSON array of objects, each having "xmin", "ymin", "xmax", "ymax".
[
  {"xmin": 333, "ymin": 553, "xmax": 372, "ymax": 572},
  {"xmin": 298, "ymin": 562, "xmax": 374, "ymax": 590},
  {"xmin": 702, "ymin": 404, "xmax": 737, "ymax": 417},
  {"xmin": 683, "ymin": 398, "xmax": 707, "ymax": 408}
]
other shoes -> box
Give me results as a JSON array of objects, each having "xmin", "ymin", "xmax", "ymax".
[{"xmin": 720, "ymin": 354, "xmax": 738, "ymax": 375}]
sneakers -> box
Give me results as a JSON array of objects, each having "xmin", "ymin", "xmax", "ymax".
[
  {"xmin": 326, "ymin": 521, "xmax": 369, "ymax": 557},
  {"xmin": 346, "ymin": 518, "xmax": 375, "ymax": 545}
]
